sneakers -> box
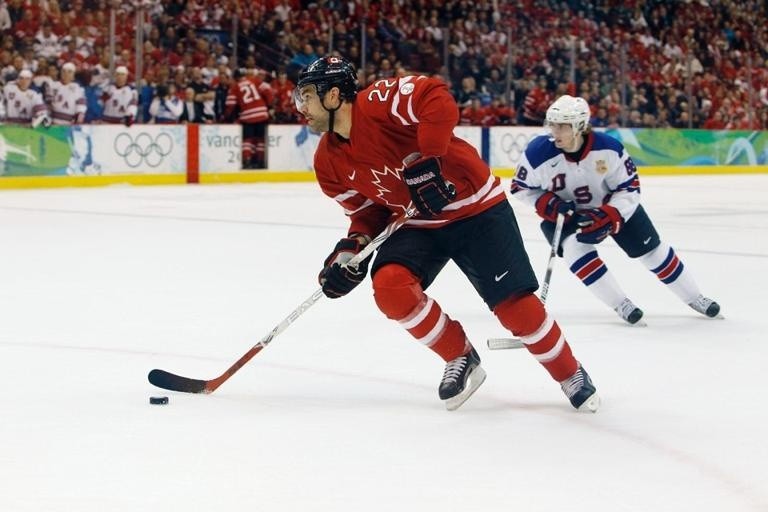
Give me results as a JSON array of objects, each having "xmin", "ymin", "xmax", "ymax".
[
  {"xmin": 438, "ymin": 341, "xmax": 481, "ymax": 400},
  {"xmin": 613, "ymin": 297, "xmax": 643, "ymax": 325},
  {"xmin": 688, "ymin": 294, "xmax": 721, "ymax": 318},
  {"xmin": 559, "ymin": 362, "xmax": 597, "ymax": 410}
]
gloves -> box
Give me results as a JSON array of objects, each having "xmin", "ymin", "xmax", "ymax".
[
  {"xmin": 535, "ymin": 192, "xmax": 575, "ymax": 223},
  {"xmin": 577, "ymin": 205, "xmax": 624, "ymax": 244},
  {"xmin": 401, "ymin": 155, "xmax": 457, "ymax": 219},
  {"xmin": 319, "ymin": 234, "xmax": 373, "ymax": 299}
]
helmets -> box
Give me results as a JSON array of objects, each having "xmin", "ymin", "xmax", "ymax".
[
  {"xmin": 541, "ymin": 94, "xmax": 592, "ymax": 146},
  {"xmin": 114, "ymin": 66, "xmax": 129, "ymax": 82},
  {"xmin": 233, "ymin": 68, "xmax": 246, "ymax": 79},
  {"xmin": 60, "ymin": 62, "xmax": 75, "ymax": 80},
  {"xmin": 294, "ymin": 55, "xmax": 358, "ymax": 112},
  {"xmin": 15, "ymin": 71, "xmax": 34, "ymax": 87}
]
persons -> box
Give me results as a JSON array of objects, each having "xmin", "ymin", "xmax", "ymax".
[
  {"xmin": 510, "ymin": 93, "xmax": 725, "ymax": 325},
  {"xmin": 294, "ymin": 55, "xmax": 601, "ymax": 413}
]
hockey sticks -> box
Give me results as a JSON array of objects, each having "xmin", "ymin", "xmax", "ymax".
[
  {"xmin": 147, "ymin": 179, "xmax": 457, "ymax": 395},
  {"xmin": 486, "ymin": 208, "xmax": 565, "ymax": 350}
]
